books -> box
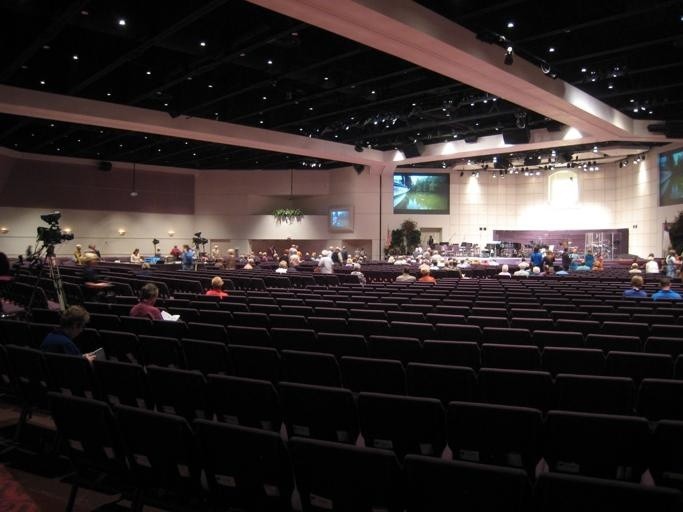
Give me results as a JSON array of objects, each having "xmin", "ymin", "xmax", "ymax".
[
  {"xmin": 93, "ymin": 347, "xmax": 107, "ymax": 360},
  {"xmin": 160, "ymin": 310, "xmax": 181, "ymax": 321}
]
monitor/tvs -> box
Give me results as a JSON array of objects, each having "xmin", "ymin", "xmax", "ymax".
[
  {"xmin": 393, "ymin": 172, "xmax": 450, "ymax": 214},
  {"xmin": 328, "ymin": 205, "xmax": 354, "ymax": 233},
  {"xmin": 659, "ymin": 147, "xmax": 683, "ymax": 207}
]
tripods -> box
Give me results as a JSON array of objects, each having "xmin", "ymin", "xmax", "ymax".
[{"xmin": 27, "ymin": 244, "xmax": 69, "ymax": 314}]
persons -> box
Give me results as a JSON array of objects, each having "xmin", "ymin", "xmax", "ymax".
[
  {"xmin": 206, "ymin": 275, "xmax": 228, "ymax": 299},
  {"xmin": 128, "ymin": 282, "xmax": 164, "ymax": 320},
  {"xmin": 623, "ymin": 246, "xmax": 682, "ymax": 300},
  {"xmin": 39, "ymin": 303, "xmax": 98, "ymax": 363},
  {"xmin": 72, "ymin": 242, "xmax": 102, "ymax": 265},
  {"xmin": 130, "ymin": 244, "xmax": 369, "ymax": 285},
  {"xmin": 388, "ymin": 235, "xmax": 604, "ymax": 286},
  {"xmin": 25, "ymin": 245, "xmax": 33, "ymax": 257}
]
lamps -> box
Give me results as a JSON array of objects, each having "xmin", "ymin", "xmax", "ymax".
[{"xmin": 489, "ymin": 153, "xmax": 649, "ymax": 179}]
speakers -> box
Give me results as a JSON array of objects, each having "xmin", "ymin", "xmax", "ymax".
[
  {"xmin": 503, "ymin": 128, "xmax": 531, "ymax": 144},
  {"xmin": 403, "ymin": 140, "xmax": 425, "ymax": 158}
]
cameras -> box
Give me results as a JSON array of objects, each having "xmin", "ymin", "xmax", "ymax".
[
  {"xmin": 37, "ymin": 212, "xmax": 75, "ymax": 245},
  {"xmin": 152, "ymin": 238, "xmax": 160, "ymax": 245},
  {"xmin": 193, "ymin": 232, "xmax": 208, "ymax": 244}
]
